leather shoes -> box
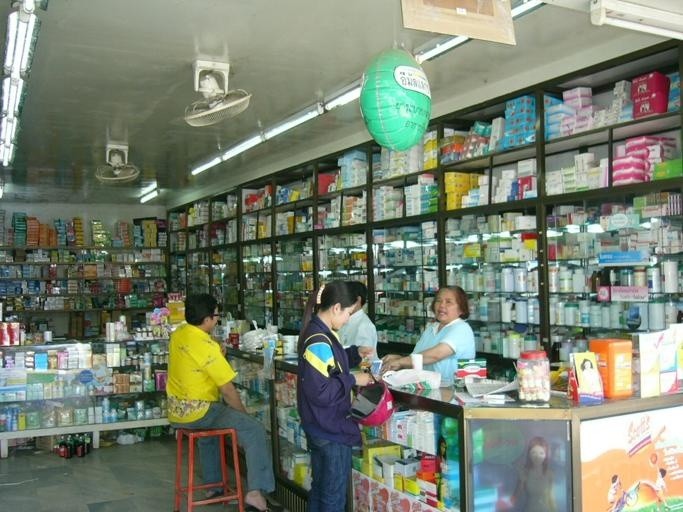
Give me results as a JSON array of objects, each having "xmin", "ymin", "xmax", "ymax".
[{"xmin": 245, "ymin": 499, "xmax": 284, "ymax": 512}]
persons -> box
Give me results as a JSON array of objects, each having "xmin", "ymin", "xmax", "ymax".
[
  {"xmin": 608, "ymin": 475, "xmax": 625, "ymax": 510},
  {"xmin": 513, "ymin": 437, "xmax": 557, "ymax": 512},
  {"xmin": 166, "ymin": 294, "xmax": 284, "ymax": 512},
  {"xmin": 297, "ymin": 280, "xmax": 375, "ymax": 512},
  {"xmin": 640, "ymin": 463, "xmax": 671, "ymax": 509},
  {"xmin": 581, "ymin": 359, "xmax": 602, "ymax": 392},
  {"xmin": 379, "ymin": 286, "xmax": 476, "ymax": 385},
  {"xmin": 435, "ymin": 437, "xmax": 447, "ymax": 503},
  {"xmin": 335, "ymin": 282, "xmax": 378, "ymax": 362}
]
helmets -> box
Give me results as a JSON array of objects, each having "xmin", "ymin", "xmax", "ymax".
[{"xmin": 352, "ymin": 381, "xmax": 396, "ymax": 427}]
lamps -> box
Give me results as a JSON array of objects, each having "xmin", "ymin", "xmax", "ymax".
[
  {"xmin": 0, "ymin": 0, "xmax": 49, "ymax": 167},
  {"xmin": 138, "ymin": 188, "xmax": 160, "ymax": 205},
  {"xmin": 590, "ymin": 0, "xmax": 683, "ymax": 41}
]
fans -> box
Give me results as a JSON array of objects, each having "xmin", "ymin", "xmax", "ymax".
[{"xmin": 96, "ymin": 144, "xmax": 140, "ymax": 185}]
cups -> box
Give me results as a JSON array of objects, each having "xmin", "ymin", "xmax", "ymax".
[{"xmin": 411, "ymin": 355, "xmax": 423, "ymax": 370}]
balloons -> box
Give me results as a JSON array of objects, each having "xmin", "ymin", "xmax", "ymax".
[{"xmin": 360, "ymin": 49, "xmax": 433, "ymax": 152}]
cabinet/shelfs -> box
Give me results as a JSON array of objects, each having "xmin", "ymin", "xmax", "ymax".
[
  {"xmin": 0, "ymin": 246, "xmax": 168, "ymax": 314},
  {"xmin": 166, "ymin": 36, "xmax": 683, "ymax": 370},
  {"xmin": 0, "ymin": 338, "xmax": 683, "ymax": 512}
]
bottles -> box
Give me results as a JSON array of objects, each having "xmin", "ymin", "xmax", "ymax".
[
  {"xmin": 126, "ymin": 351, "xmax": 169, "ymax": 366},
  {"xmin": 516, "ymin": 350, "xmax": 551, "ymax": 401},
  {"xmin": 11, "ymin": 415, "xmax": 18, "ymax": 431},
  {"xmin": 447, "ymin": 263, "xmax": 678, "ymax": 363},
  {"xmin": 59, "ymin": 433, "xmax": 90, "ymax": 459},
  {"xmin": 101, "ymin": 395, "xmax": 117, "ymax": 423},
  {"xmin": 136, "ymin": 327, "xmax": 153, "ymax": 339},
  {"xmin": 282, "ymin": 335, "xmax": 294, "ymax": 354}
]
